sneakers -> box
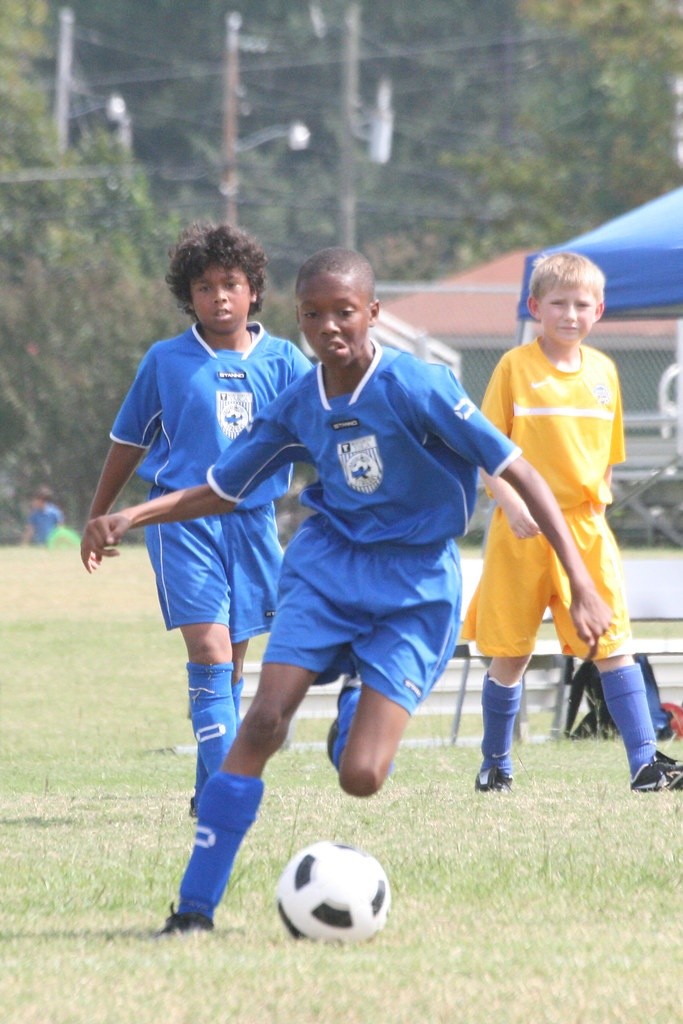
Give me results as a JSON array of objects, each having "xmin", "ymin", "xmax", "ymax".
[
  {"xmin": 631, "ymin": 751, "xmax": 683, "ymax": 791},
  {"xmin": 476, "ymin": 766, "xmax": 512, "ymax": 793},
  {"xmin": 326, "ymin": 670, "xmax": 363, "ymax": 763}
]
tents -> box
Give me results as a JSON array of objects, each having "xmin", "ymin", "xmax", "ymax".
[{"xmin": 436, "ymin": 187, "xmax": 683, "ymax": 746}]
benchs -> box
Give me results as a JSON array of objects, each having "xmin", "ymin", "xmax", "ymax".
[{"xmin": 443, "ymin": 555, "xmax": 682, "ymax": 755}]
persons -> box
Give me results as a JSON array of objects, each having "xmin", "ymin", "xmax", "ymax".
[
  {"xmin": 15, "ymin": 485, "xmax": 69, "ymax": 550},
  {"xmin": 472, "ymin": 249, "xmax": 681, "ymax": 801},
  {"xmin": 83, "ymin": 219, "xmax": 323, "ymax": 814},
  {"xmin": 77, "ymin": 243, "xmax": 619, "ymax": 942}
]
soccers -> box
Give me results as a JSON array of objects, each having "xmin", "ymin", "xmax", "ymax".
[{"xmin": 275, "ymin": 840, "xmax": 391, "ymax": 945}]
